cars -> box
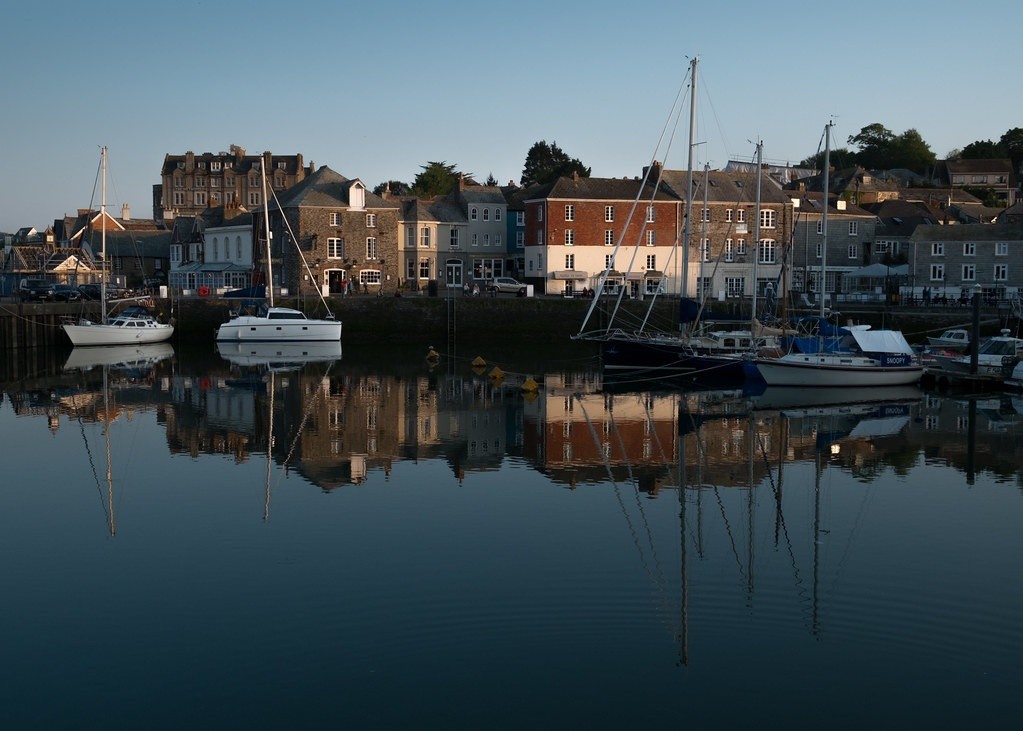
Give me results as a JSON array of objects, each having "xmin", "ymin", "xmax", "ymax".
[
  {"xmin": 52, "ymin": 283, "xmax": 81, "ymax": 303},
  {"xmin": 101, "ymin": 282, "xmax": 136, "ymax": 299}
]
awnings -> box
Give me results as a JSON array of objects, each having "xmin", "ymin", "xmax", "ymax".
[{"xmin": 554, "ymin": 271, "xmax": 588, "ymax": 279}]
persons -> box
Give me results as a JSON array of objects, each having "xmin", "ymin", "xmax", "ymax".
[
  {"xmin": 919, "ymin": 286, "xmax": 931, "ymax": 306},
  {"xmin": 472, "ymin": 282, "xmax": 480, "ymax": 297},
  {"xmin": 364, "ymin": 276, "xmax": 369, "ymax": 294},
  {"xmin": 463, "ymin": 282, "xmax": 470, "ymax": 296},
  {"xmin": 341, "ymin": 276, "xmax": 347, "ymax": 298},
  {"xmin": 347, "ymin": 278, "xmax": 353, "ymax": 297},
  {"xmin": 941, "ymin": 295, "xmax": 947, "ymax": 307},
  {"xmin": 934, "ymin": 293, "xmax": 939, "ymax": 307},
  {"xmin": 581, "ymin": 287, "xmax": 595, "ymax": 299},
  {"xmin": 377, "ymin": 289, "xmax": 383, "ymax": 298}
]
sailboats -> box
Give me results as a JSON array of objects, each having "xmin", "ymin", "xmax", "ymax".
[
  {"xmin": 558, "ymin": 52, "xmax": 1022, "ymax": 403},
  {"xmin": 211, "ymin": 334, "xmax": 343, "ymax": 522},
  {"xmin": 59, "ymin": 144, "xmax": 175, "ymax": 347},
  {"xmin": 738, "ymin": 380, "xmax": 934, "ymax": 646},
  {"xmin": 211, "ymin": 153, "xmax": 343, "ymax": 343},
  {"xmin": 60, "ymin": 339, "xmax": 176, "ymax": 539}
]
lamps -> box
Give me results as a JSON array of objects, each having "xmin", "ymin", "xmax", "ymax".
[
  {"xmin": 352, "ymin": 259, "xmax": 357, "ymax": 265},
  {"xmin": 315, "ymin": 258, "xmax": 321, "ymax": 264},
  {"xmin": 344, "ymin": 259, "xmax": 349, "ymax": 263},
  {"xmin": 380, "ymin": 259, "xmax": 385, "ymax": 264}
]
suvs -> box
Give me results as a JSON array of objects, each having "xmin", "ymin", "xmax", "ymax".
[
  {"xmin": 486, "ymin": 276, "xmax": 528, "ymax": 298},
  {"xmin": 18, "ymin": 277, "xmax": 55, "ymax": 303},
  {"xmin": 76, "ymin": 283, "xmax": 111, "ymax": 301}
]
